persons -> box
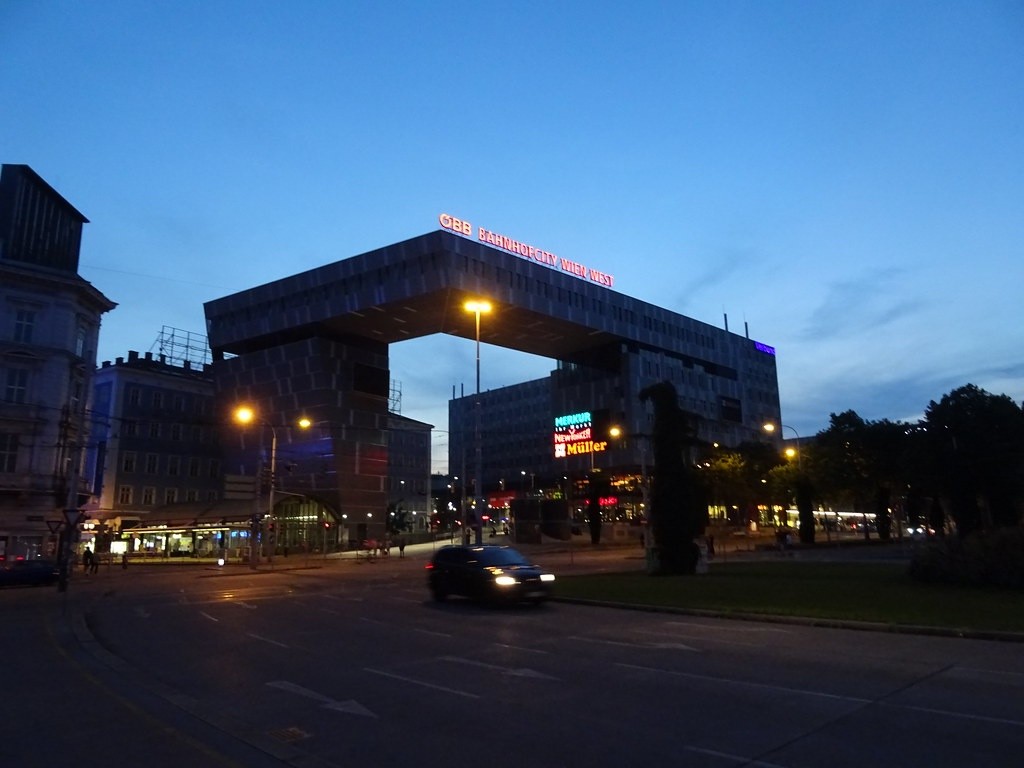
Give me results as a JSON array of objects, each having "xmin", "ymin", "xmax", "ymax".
[{"xmin": 82, "ymin": 546, "xmax": 101, "ymax": 576}]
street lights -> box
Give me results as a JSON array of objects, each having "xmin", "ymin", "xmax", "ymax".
[
  {"xmin": 237, "ymin": 408, "xmax": 311, "ymax": 570},
  {"xmin": 609, "ymin": 425, "xmax": 648, "ymax": 558},
  {"xmin": 465, "ymin": 300, "xmax": 493, "ymax": 547},
  {"xmin": 764, "ymin": 424, "xmax": 801, "ymax": 470}
]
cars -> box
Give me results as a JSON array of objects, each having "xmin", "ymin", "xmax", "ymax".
[{"xmin": 425, "ymin": 545, "xmax": 554, "ymax": 610}]
